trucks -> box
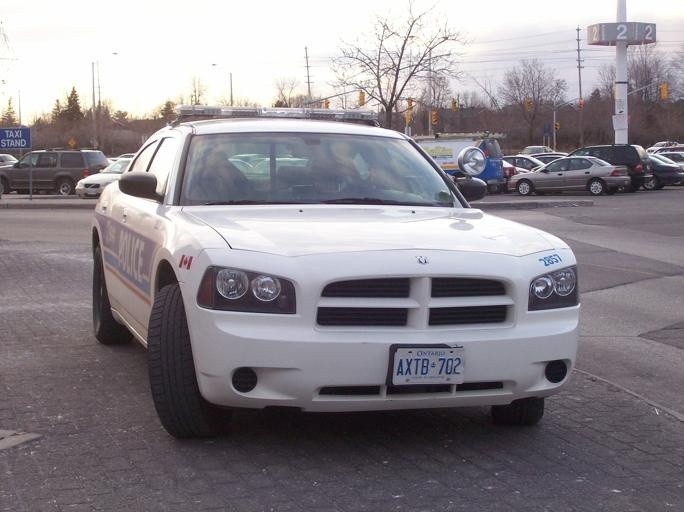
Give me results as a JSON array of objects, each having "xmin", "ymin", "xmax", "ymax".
[{"xmin": 413, "ymin": 132, "xmax": 503, "ymax": 195}]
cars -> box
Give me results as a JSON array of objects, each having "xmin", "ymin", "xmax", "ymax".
[
  {"xmin": 501, "ymin": 138, "xmax": 684, "ymax": 193},
  {"xmin": 0, "ymin": 146, "xmax": 136, "ymax": 199},
  {"xmin": 90, "ymin": 104, "xmax": 581, "ymax": 439},
  {"xmin": 227, "ymin": 152, "xmax": 309, "ymax": 176}
]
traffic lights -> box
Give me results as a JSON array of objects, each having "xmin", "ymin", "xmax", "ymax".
[
  {"xmin": 432, "ymin": 110, "xmax": 438, "ymax": 126},
  {"xmin": 556, "ymin": 122, "xmax": 560, "ymax": 133},
  {"xmin": 326, "ymin": 100, "xmax": 330, "ymax": 108},
  {"xmin": 454, "ymin": 102, "xmax": 457, "ymax": 112},
  {"xmin": 528, "ymin": 100, "xmax": 532, "ymax": 111}
]
